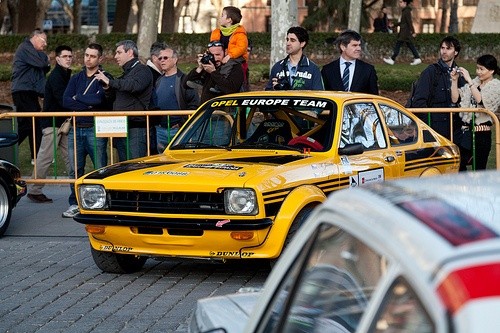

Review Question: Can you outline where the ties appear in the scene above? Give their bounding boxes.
[341,62,352,92]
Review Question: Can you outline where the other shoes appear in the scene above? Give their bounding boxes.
[31,159,55,165]
[27,192,53,202]
[409,58,422,65]
[383,57,395,65]
[186,78,204,90]
[62,204,80,218]
[209,84,222,92]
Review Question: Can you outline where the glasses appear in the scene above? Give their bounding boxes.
[207,41,223,47]
[158,56,169,60]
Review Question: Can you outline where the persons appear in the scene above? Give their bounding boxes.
[152,48,199,154]
[27,45,74,204]
[451,54,500,171]
[321,30,378,95]
[373,6,388,33]
[62,43,117,218]
[183,39,245,147]
[383,0,422,66]
[94,39,154,162]
[263,26,325,120]
[146,41,170,81]
[404,35,470,172]
[209,6,252,94]
[9,28,56,166]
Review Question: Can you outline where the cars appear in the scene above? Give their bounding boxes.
[188,168,500,333]
[0,131,27,239]
[72,89,461,273]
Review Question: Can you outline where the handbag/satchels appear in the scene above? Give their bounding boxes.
[57,118,73,137]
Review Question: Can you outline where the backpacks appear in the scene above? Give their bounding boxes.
[405,62,449,107]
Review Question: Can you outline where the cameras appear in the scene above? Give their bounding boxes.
[201,53,215,65]
[455,68,463,73]
